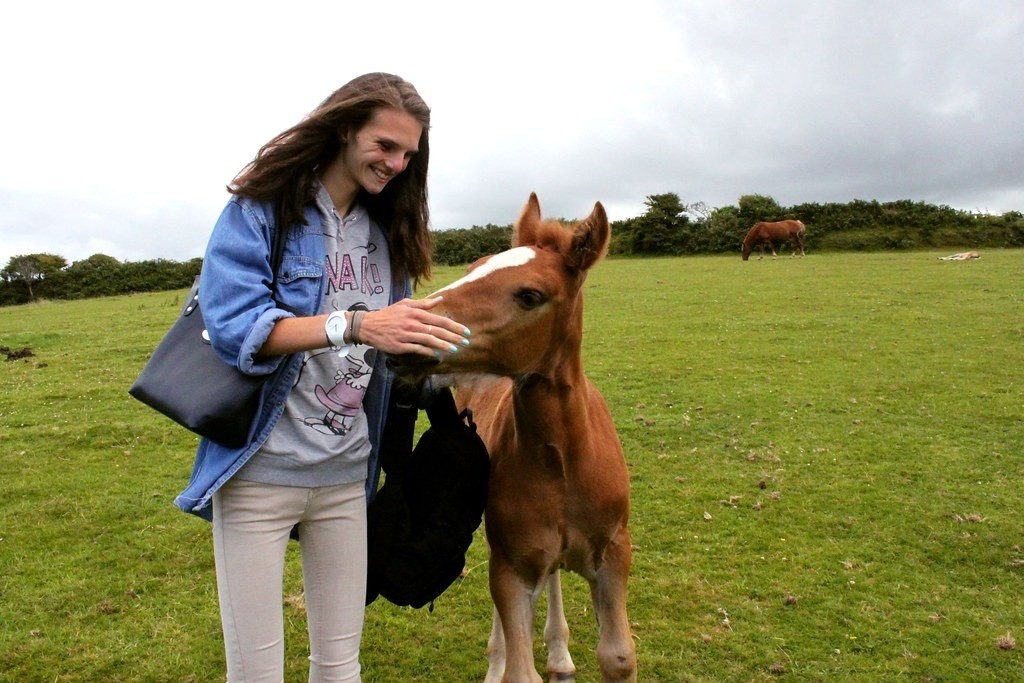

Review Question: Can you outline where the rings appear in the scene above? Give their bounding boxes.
[428,324,432,334]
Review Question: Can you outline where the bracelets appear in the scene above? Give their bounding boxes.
[348,310,366,347]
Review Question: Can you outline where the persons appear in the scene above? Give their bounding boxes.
[173,72,471,682]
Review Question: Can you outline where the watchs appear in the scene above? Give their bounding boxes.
[325,310,352,348]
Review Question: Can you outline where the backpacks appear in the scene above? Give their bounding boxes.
[367,374,490,610]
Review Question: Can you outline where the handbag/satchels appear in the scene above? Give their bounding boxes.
[127,198,310,451]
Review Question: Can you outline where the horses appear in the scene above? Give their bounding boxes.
[739,219,807,261]
[384,193,638,683]
